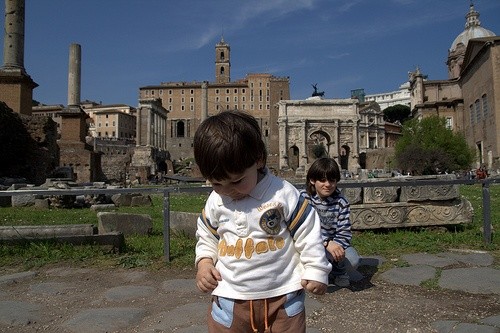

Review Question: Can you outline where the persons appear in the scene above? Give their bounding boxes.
[300,158,359,286]
[454,166,473,180]
[194,110,332,333]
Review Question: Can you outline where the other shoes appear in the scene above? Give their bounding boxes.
[336,273,350,286]
